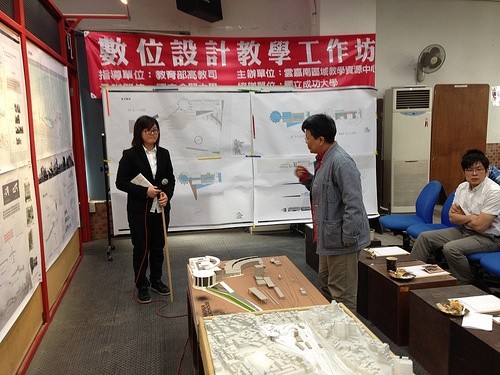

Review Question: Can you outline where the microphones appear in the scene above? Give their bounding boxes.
[146,178,169,199]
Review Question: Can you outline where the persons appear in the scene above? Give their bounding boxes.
[39,155,71,179]
[116,115,175,302]
[408,149,500,284]
[294,113,372,316]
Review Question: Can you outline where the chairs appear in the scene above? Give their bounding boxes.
[406,190,458,269]
[378,181,443,250]
[467,251,500,279]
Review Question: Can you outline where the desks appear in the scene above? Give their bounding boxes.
[186,254,331,375]
[304,223,381,274]
[409,284,499,375]
[357,246,456,346]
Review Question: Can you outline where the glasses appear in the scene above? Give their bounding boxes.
[466,167,484,173]
[145,129,160,134]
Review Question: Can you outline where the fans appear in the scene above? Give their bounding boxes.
[416,44,447,82]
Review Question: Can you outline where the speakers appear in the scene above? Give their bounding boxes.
[175,0,223,23]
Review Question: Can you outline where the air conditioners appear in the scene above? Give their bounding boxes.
[382,86,433,214]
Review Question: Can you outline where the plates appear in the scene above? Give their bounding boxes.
[439,304,470,316]
[390,273,416,281]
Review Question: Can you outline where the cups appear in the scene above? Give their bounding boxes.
[370,229,375,240]
[386,257,398,272]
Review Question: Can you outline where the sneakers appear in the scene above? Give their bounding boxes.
[151,283,171,295]
[137,287,152,304]
